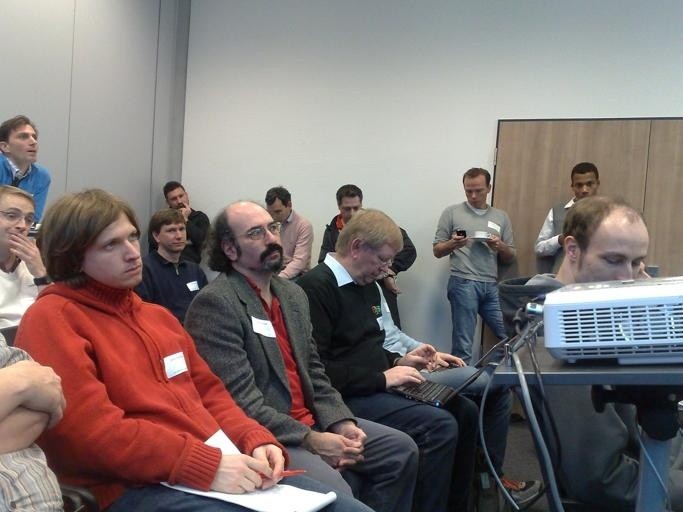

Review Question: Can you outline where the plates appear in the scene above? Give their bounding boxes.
[468,237,493,243]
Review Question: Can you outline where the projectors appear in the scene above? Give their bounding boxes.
[542,276,683,366]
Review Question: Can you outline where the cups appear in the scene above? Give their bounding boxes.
[474,231,491,238]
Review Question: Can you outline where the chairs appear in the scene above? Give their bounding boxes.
[0,326,100,512]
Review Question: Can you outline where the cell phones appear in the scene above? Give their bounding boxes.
[455,229,467,238]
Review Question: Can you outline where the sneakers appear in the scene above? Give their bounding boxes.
[498,475,545,510]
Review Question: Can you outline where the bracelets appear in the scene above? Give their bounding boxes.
[34,274,52,285]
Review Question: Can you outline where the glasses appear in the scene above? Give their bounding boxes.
[234,220,281,241]
[0,211,35,229]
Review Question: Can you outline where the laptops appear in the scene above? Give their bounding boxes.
[418,336,510,374]
[385,364,488,407]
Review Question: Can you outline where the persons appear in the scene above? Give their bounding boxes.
[513,195,683,512]
[316,185,418,330]
[12,189,376,511]
[148,181,211,266]
[1,115,51,222]
[294,208,482,512]
[1,331,70,511]
[433,168,516,366]
[134,209,209,325]
[534,162,601,273]
[264,186,313,280]
[375,281,549,511]
[183,200,418,512]
[1,185,54,328]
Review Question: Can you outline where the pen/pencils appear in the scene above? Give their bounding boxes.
[257,469,307,478]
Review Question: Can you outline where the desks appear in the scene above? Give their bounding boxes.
[493,336,683,511]
